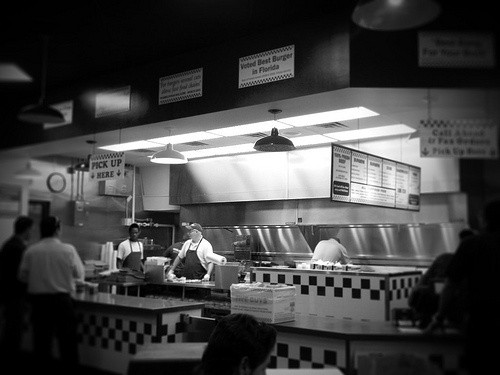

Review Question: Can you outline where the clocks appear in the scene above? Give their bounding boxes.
[47,173,67,193]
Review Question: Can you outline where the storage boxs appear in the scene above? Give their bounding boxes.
[215,265,239,289]
[230,282,296,324]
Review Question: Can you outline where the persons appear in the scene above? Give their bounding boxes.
[310,237,354,266]
[116,223,145,273]
[165,222,214,281]
[0,217,35,375]
[408,199,500,375]
[194,313,278,375]
[19,215,86,375]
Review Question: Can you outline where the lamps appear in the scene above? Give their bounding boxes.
[150,126,188,164]
[253,109,296,152]
[12,146,43,180]
[17,57,65,125]
[351,0,440,30]
[75,140,97,171]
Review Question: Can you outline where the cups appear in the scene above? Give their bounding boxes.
[100,241,117,294]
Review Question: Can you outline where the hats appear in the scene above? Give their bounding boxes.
[186,223,204,232]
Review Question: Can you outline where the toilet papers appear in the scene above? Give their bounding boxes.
[206,253,227,266]
